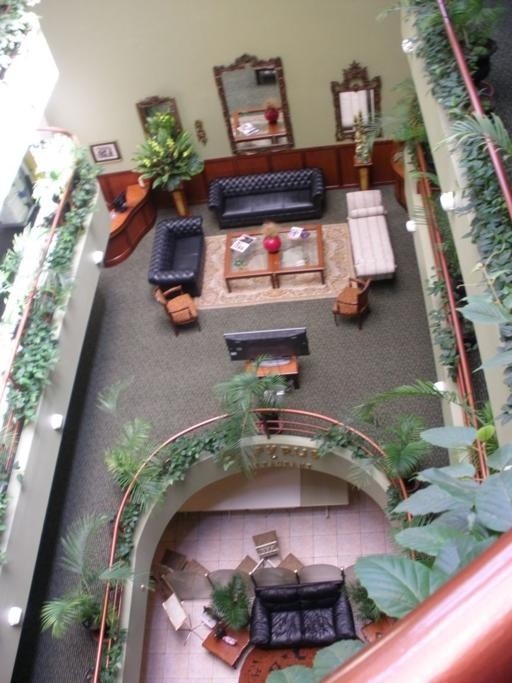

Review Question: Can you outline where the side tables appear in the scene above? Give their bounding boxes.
[201,620,250,670]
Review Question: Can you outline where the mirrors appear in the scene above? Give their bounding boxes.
[213,55,305,149]
[332,60,381,141]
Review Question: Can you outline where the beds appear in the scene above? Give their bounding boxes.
[346,189,397,283]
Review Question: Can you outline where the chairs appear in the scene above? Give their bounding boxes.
[152,284,201,335]
[332,276,373,328]
[157,530,305,577]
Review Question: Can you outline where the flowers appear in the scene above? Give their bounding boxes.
[132,111,204,191]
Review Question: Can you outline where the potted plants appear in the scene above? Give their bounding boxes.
[363,416,431,490]
[38,508,146,640]
[214,353,286,481]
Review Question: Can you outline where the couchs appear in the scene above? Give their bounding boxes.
[250,580,356,649]
[148,216,205,297]
[208,167,325,229]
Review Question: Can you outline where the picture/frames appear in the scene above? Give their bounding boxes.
[90,142,120,163]
[136,96,183,147]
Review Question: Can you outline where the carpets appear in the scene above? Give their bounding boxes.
[192,223,357,310]
[239,645,324,683]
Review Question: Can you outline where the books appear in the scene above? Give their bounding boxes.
[237,123,260,136]
[230,234,256,252]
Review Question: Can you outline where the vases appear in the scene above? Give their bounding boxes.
[263,236,282,253]
[173,189,189,218]
[265,109,278,124]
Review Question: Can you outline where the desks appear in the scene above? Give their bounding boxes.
[245,356,300,390]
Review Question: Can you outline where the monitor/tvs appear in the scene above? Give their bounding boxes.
[113,192,128,213]
[224,327,310,367]
